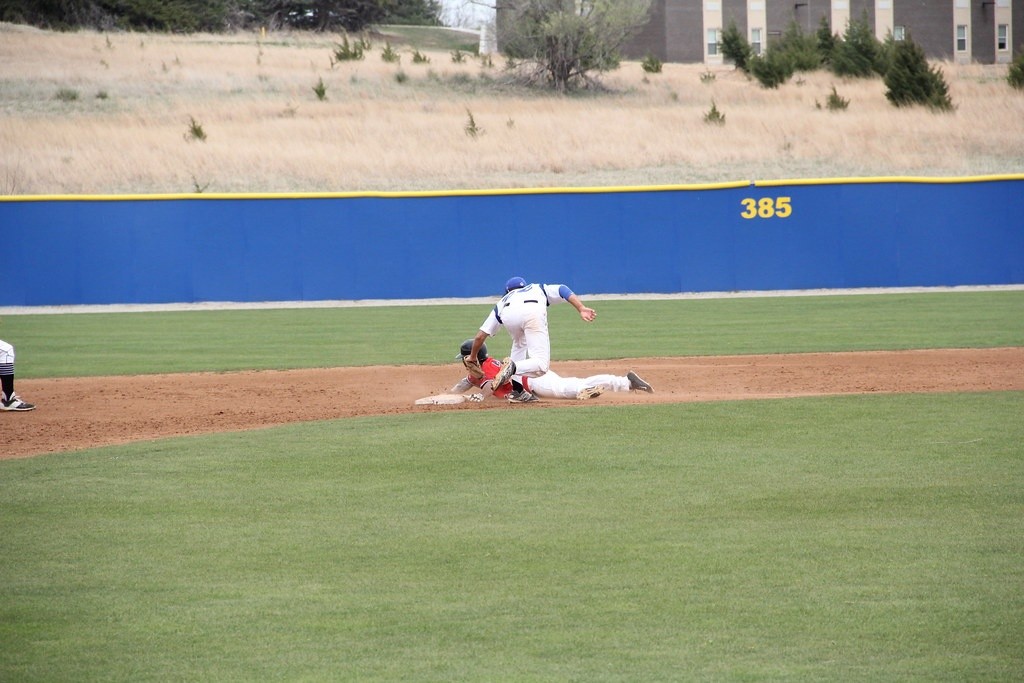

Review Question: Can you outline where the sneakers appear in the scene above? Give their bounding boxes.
[508,389,540,403]
[578,384,606,401]
[1,392,36,411]
[626,370,653,393]
[491,358,516,390]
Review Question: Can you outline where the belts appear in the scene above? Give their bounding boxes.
[502,300,542,305]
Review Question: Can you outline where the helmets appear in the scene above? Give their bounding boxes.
[502,277,527,297]
[455,339,489,361]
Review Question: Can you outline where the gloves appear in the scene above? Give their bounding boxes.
[463,393,484,402]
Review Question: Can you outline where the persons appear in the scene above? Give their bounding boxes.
[465,276,597,405]
[441,339,656,404]
[1,338,37,413]
